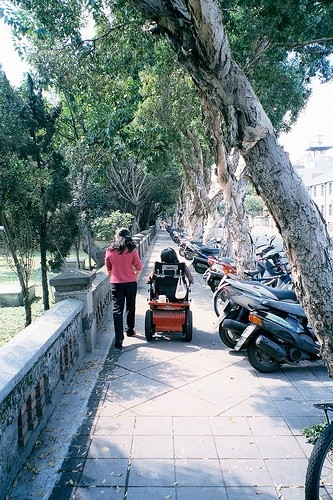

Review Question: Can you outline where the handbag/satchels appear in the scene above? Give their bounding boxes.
[174,278,187,299]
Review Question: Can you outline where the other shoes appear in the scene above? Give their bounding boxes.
[126,330,136,337]
[113,342,126,349]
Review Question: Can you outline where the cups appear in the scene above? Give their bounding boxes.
[159,295,169,303]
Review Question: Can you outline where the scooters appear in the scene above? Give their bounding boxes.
[164,224,300,351]
[234,299,322,373]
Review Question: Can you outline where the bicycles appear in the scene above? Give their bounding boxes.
[304,402,333,500]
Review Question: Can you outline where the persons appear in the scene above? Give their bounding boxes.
[105,228,142,348]
[147,247,194,286]
